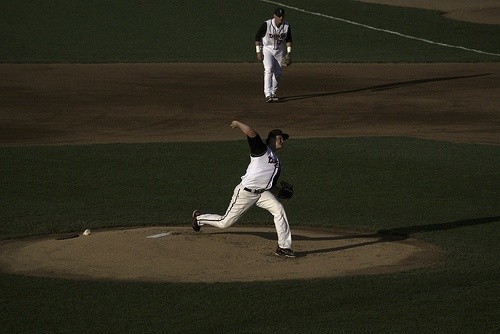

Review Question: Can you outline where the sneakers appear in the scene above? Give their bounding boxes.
[191,209,201,232]
[276,247,296,259]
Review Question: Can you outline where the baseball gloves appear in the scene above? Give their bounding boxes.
[272,181,294,199]
[281,54,292,67]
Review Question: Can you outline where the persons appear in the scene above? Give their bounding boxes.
[192,120,295,257]
[255,8,293,102]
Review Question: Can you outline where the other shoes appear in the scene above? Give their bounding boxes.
[264,96,279,102]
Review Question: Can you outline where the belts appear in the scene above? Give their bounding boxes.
[244,187,266,194]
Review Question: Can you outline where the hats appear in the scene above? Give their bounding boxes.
[274,8,285,16]
[268,129,290,142]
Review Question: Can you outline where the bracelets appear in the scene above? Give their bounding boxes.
[256,46,260,53]
[287,47,291,53]
